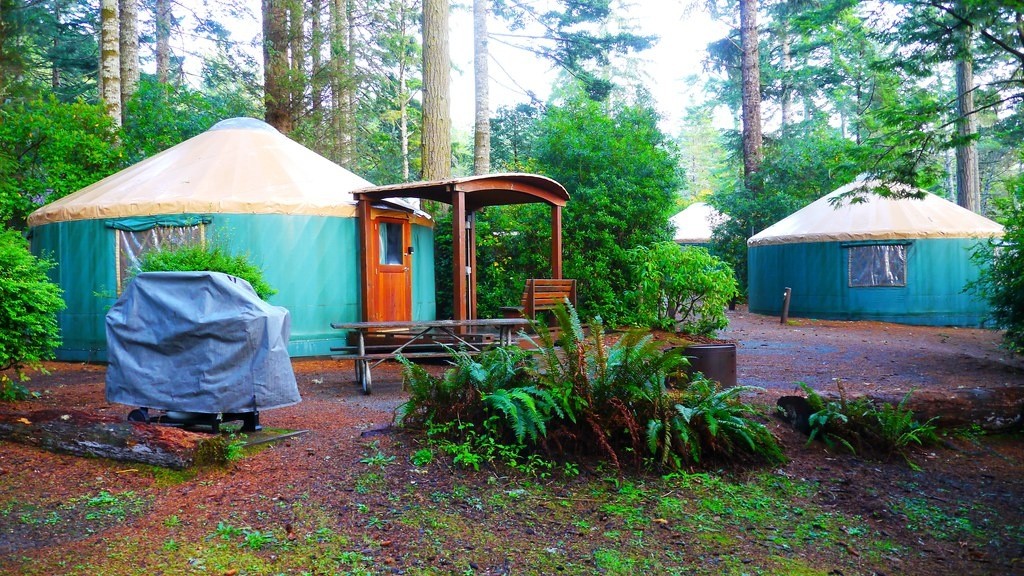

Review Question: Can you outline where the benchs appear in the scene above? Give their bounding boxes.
[329,341,520,383]
[497,277,577,334]
[331,351,491,396]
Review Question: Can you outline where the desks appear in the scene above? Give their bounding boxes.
[330,318,538,389]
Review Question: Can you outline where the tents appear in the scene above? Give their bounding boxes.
[27,117,438,360]
[667,202,744,280]
[747,180,1014,330]
